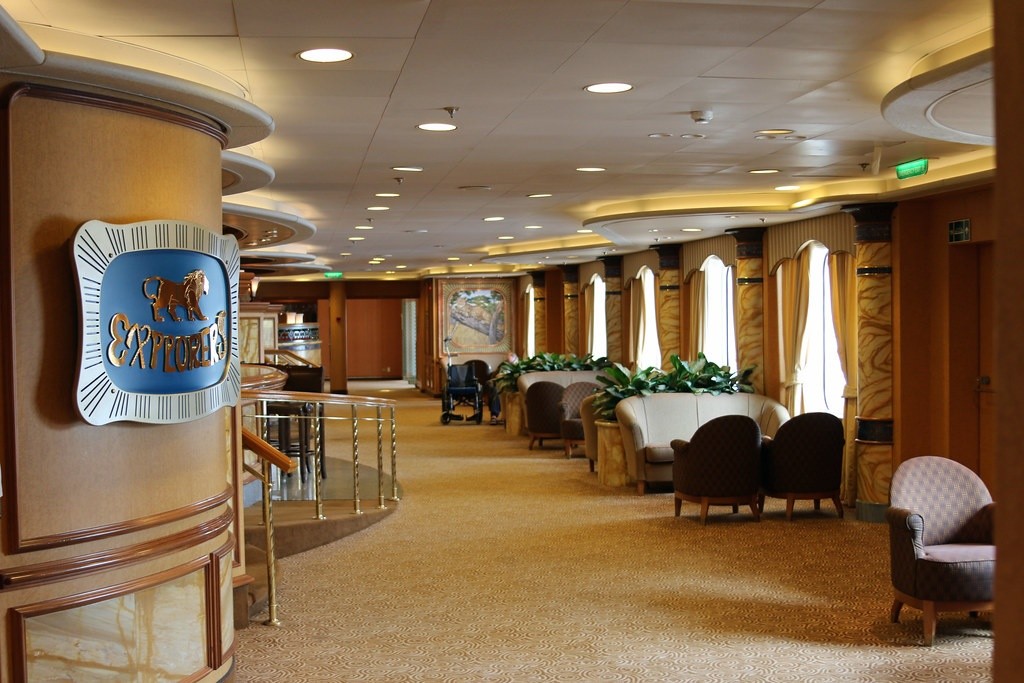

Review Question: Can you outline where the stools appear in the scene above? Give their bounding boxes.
[267,366,327,483]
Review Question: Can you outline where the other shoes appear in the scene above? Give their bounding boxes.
[489,418,497,425]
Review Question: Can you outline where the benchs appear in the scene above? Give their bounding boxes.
[615,393,791,497]
[517,371,616,429]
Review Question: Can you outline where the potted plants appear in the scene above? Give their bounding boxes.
[592,363,667,488]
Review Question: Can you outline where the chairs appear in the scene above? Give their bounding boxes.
[580,391,607,473]
[464,360,491,380]
[887,456,996,647]
[557,382,602,459]
[525,381,565,451]
[758,412,846,523]
[670,415,762,527]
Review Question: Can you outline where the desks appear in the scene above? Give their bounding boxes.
[240,364,288,392]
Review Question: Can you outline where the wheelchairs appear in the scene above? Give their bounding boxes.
[440,362,484,425]
